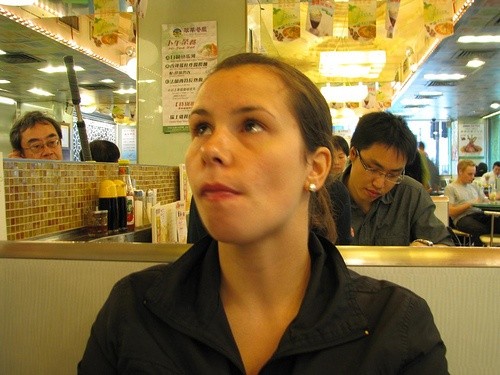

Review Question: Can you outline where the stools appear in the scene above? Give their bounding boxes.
[450,229,475,247]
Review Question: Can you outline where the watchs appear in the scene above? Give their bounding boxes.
[413,239,433,247]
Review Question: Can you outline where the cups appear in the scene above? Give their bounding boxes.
[88,210,108,236]
[310,9,322,31]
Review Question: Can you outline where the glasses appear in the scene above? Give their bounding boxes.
[21,139,60,153]
[356,146,405,184]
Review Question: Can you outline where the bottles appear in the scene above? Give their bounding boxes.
[118,159,135,229]
[134,189,154,227]
[112,179,128,231]
[97,179,119,233]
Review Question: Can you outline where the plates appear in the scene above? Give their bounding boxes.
[282,25,300,41]
[196,50,217,61]
[357,23,376,40]
[433,21,454,37]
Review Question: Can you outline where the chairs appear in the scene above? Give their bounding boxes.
[479,210,500,248]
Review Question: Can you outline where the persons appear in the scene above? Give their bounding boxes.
[318,112,456,249]
[443,160,500,246]
[328,133,349,178]
[79,139,122,163]
[74,55,450,375]
[8,111,64,164]
[404,134,443,196]
